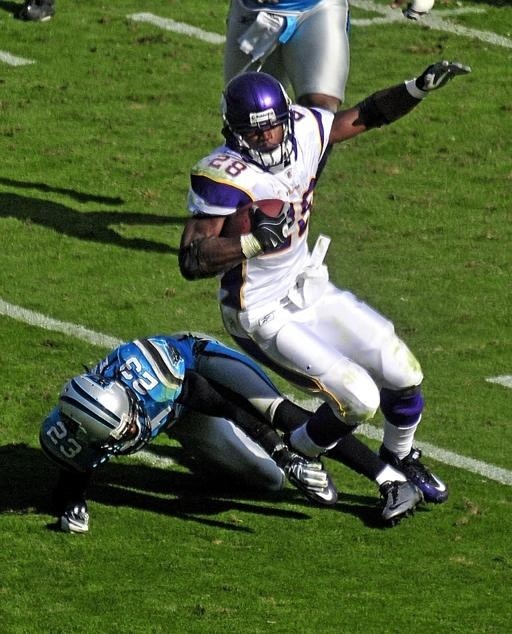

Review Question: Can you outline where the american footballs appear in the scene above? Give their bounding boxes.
[219,199,285,237]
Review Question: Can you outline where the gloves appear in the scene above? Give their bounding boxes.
[247,200,310,257]
[415,59,474,92]
[59,504,89,534]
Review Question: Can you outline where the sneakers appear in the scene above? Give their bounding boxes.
[378,440,449,503]
[273,429,339,506]
[379,479,424,523]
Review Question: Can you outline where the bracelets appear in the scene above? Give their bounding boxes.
[404,77,429,100]
[238,232,262,259]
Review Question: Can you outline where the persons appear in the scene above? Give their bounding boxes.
[220,0,439,186]
[178,58,473,504]
[44,331,422,535]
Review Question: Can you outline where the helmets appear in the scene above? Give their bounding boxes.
[57,373,138,447]
[219,69,293,138]
